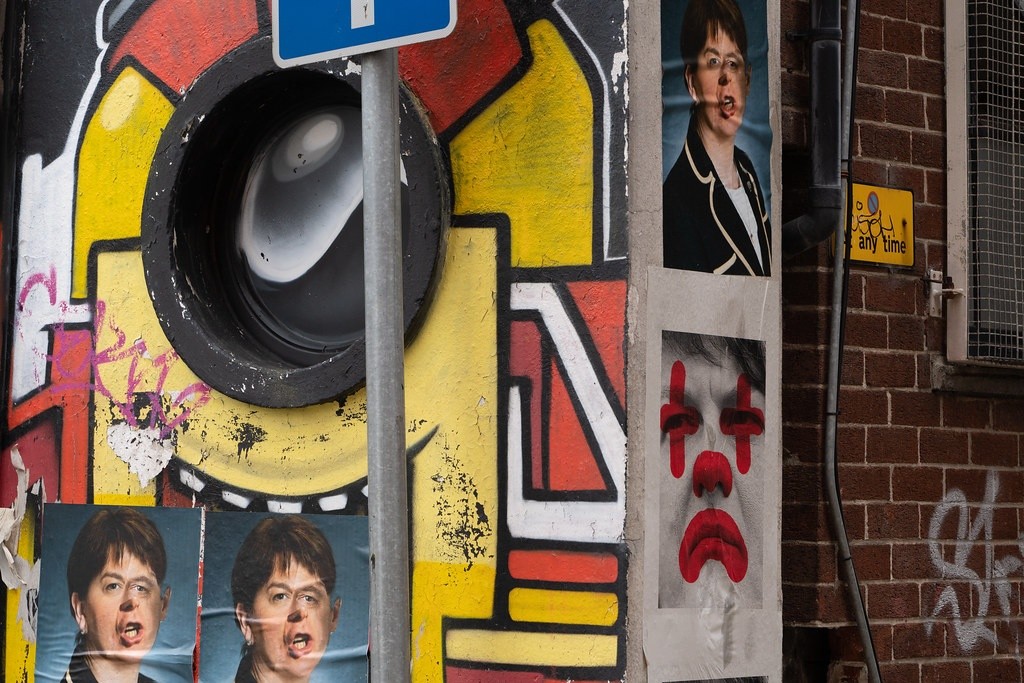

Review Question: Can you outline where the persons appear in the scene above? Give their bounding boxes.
[230,514,341,683]
[660,327,763,610]
[663,1,771,277]
[59,505,172,683]
